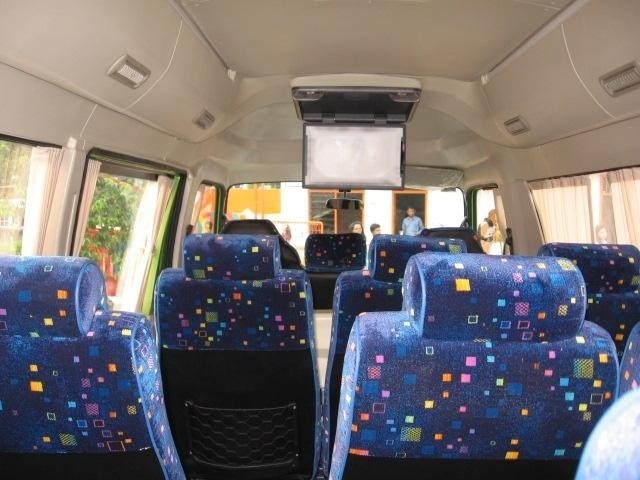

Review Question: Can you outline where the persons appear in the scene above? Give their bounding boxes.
[401,207,425,236]
[348,221,368,271]
[478,208,504,256]
[370,223,381,237]
[595,224,610,244]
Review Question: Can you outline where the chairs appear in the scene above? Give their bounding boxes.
[1,221,638,478]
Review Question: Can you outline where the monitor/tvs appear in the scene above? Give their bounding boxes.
[301,122,407,191]
